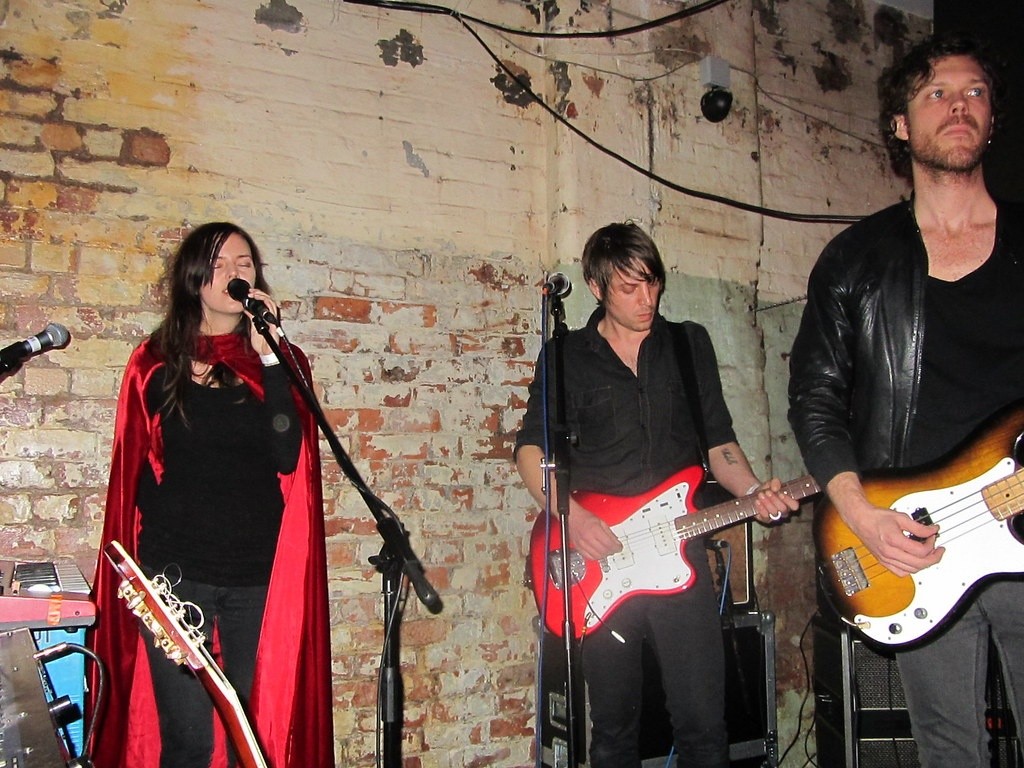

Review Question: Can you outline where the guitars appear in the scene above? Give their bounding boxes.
[527,462,824,642]
[103,538,274,768]
[809,405,1024,657]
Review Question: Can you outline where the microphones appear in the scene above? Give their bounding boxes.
[0,323,69,363]
[542,272,570,294]
[227,278,276,324]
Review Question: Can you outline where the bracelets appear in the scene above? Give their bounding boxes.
[259,352,280,367]
[744,483,760,496]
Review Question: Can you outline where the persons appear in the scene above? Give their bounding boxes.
[514,219,799,768]
[786,30,1024,768]
[82,222,335,768]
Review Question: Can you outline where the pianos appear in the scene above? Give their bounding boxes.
[0,557,98,636]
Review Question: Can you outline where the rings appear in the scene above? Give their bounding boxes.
[768,510,784,520]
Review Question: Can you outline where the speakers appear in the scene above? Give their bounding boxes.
[813,616,1024,768]
